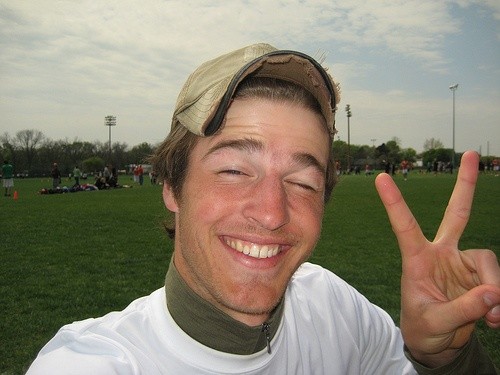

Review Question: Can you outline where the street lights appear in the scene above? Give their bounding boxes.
[449,83,459,167]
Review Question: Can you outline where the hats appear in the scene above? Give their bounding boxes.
[173,42,345,149]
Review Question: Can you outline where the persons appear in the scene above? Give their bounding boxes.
[49,162,158,189]
[21,41,500,375]
[1,159,14,197]
[334,157,500,181]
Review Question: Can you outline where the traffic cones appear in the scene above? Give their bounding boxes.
[14,191,18,200]
